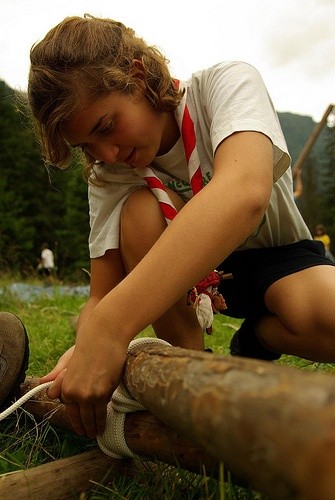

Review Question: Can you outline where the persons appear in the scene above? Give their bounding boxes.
[26,13,335,438]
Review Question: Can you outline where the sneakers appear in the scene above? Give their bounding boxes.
[1,312,29,434]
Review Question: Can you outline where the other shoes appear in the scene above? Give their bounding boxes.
[231,312,283,361]
